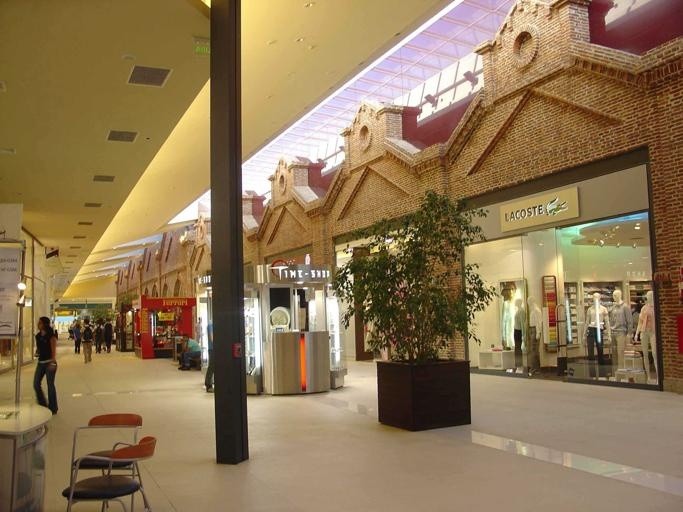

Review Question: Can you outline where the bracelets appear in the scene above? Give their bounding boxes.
[48,363,57,366]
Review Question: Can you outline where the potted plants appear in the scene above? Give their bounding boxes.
[329,186,494,433]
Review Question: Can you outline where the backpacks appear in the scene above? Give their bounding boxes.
[82,325,93,341]
[95,324,102,339]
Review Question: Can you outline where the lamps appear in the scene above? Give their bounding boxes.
[16,295,25,307]
[19,277,26,291]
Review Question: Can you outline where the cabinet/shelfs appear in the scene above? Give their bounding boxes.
[564,277,653,345]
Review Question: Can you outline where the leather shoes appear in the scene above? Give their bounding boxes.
[181,366,191,370]
[206,385,213,393]
[178,366,184,369]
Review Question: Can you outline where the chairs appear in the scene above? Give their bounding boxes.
[60,435,155,511]
[67,412,149,510]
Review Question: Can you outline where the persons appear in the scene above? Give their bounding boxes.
[32,316,58,415]
[73,323,82,354]
[103,317,114,353]
[178,334,201,370]
[582,291,612,382]
[633,291,659,385]
[512,298,526,373]
[630,301,643,331]
[80,318,96,364]
[202,321,216,393]
[194,316,205,344]
[608,288,635,379]
[92,320,104,354]
[523,296,542,376]
